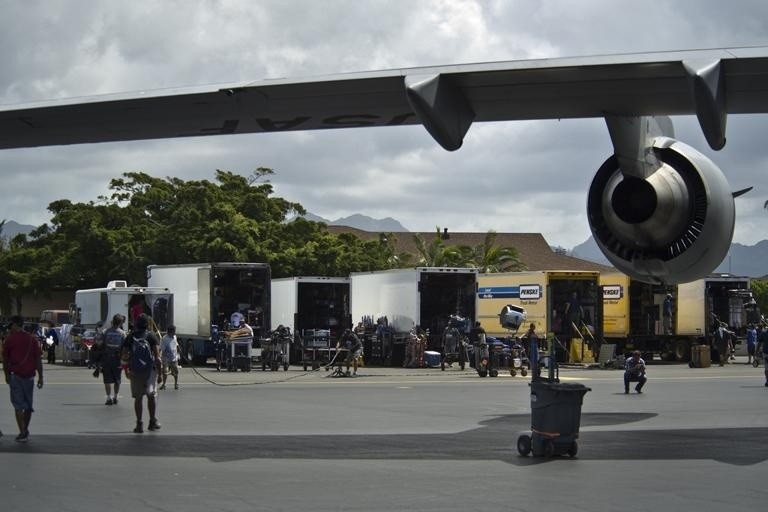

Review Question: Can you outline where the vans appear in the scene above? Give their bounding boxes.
[41,309,75,325]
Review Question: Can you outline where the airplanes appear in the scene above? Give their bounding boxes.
[0,44,768,290]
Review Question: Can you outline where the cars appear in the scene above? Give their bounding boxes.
[0,322,48,356]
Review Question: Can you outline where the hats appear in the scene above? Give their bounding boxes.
[7,313,25,325]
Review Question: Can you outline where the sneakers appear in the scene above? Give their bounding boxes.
[15,431,29,441]
[133,420,144,433]
[112,398,118,404]
[624,389,630,396]
[175,383,179,390]
[635,387,642,393]
[345,370,359,377]
[148,417,161,431]
[160,383,167,390]
[105,399,112,405]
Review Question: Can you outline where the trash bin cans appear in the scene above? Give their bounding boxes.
[516,378,592,459]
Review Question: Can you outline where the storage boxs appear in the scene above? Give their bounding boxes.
[422,348,443,367]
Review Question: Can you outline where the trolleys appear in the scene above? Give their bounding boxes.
[752,339,765,368]
[217,336,252,373]
[516,332,591,459]
[261,337,289,373]
[303,326,331,373]
[481,342,532,378]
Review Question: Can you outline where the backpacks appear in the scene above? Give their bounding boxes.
[129,331,156,371]
[103,327,124,361]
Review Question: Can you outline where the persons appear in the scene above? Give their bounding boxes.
[130,299,143,324]
[158,324,183,390]
[70,319,86,336]
[622,349,647,393]
[3,315,43,443]
[663,293,673,335]
[562,289,584,337]
[27,323,58,364]
[96,315,126,406]
[122,311,164,432]
[552,309,562,332]
[718,317,768,386]
